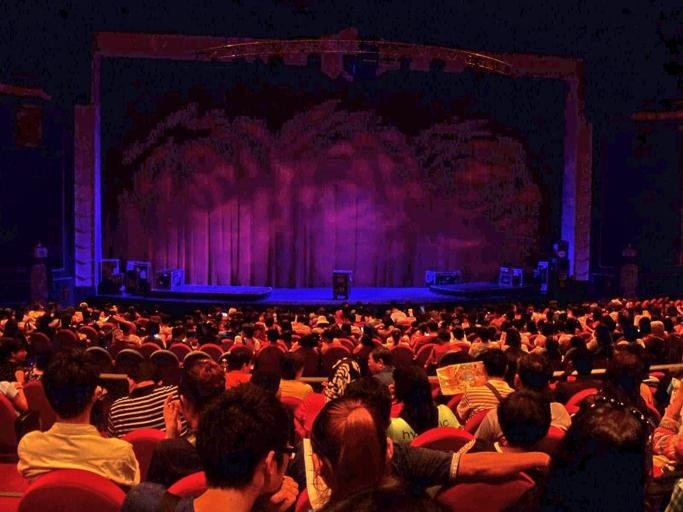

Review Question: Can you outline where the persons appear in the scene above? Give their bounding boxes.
[562,352,606,403]
[15,349,140,490]
[543,396,651,511]
[381,367,463,437]
[479,355,564,446]
[295,355,365,432]
[457,352,520,422]
[1,383,32,422]
[344,376,414,444]
[217,349,257,389]
[275,353,320,400]
[570,345,663,431]
[650,375,683,479]
[418,293,683,373]
[0,338,29,393]
[107,360,190,438]
[124,384,300,512]
[252,367,278,400]
[306,396,451,511]
[146,354,226,486]
[365,352,402,375]
[290,375,553,492]
[472,395,564,496]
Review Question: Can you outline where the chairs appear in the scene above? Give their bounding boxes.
[0,464,37,512]
[24,379,57,433]
[102,374,129,398]
[16,468,125,512]
[1,391,37,454]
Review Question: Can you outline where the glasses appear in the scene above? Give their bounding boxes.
[280,443,298,461]
